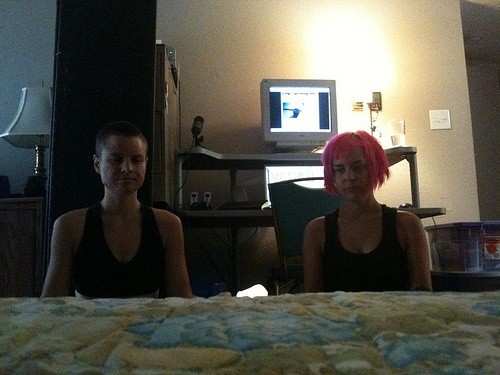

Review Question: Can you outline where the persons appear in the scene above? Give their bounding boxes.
[38,121,196,299]
[300,130,435,292]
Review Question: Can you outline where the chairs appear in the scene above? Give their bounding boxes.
[268,177,343,295]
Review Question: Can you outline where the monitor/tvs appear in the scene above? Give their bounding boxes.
[260,79,338,153]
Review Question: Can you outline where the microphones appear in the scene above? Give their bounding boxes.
[191,116,204,137]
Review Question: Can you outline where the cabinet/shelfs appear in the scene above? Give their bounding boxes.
[1,198,47,299]
[175,145,447,300]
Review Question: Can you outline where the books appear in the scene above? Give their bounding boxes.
[218,199,271,211]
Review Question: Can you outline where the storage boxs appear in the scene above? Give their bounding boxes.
[424,220,499,276]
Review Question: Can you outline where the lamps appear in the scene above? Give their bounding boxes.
[1,80,52,196]
